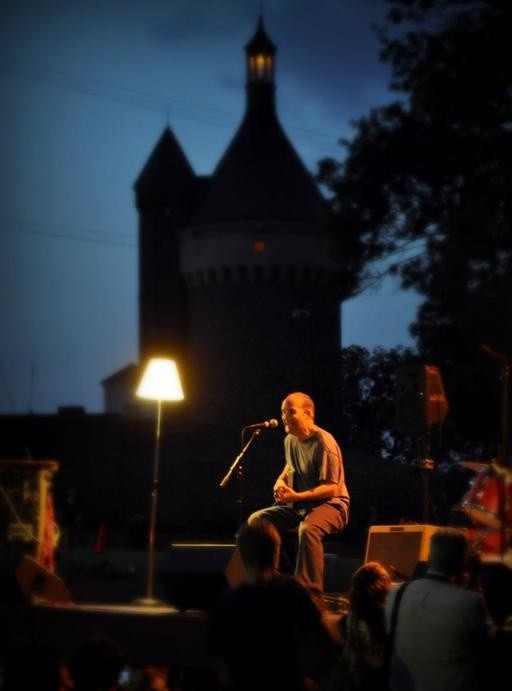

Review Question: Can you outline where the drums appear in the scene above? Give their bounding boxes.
[459,467,512,529]
[462,526,512,562]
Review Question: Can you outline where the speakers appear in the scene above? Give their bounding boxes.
[172,545,252,612]
[14,555,70,607]
[395,365,449,434]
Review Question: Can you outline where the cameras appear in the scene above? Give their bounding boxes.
[117,668,143,691]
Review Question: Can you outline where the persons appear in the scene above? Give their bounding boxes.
[0,519,512,691]
[246,391,350,592]
[461,448,512,568]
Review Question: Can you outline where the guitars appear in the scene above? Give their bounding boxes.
[274,468,294,509]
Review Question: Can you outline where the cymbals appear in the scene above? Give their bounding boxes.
[457,461,489,473]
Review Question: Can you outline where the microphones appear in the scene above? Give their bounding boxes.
[249,418,279,429]
